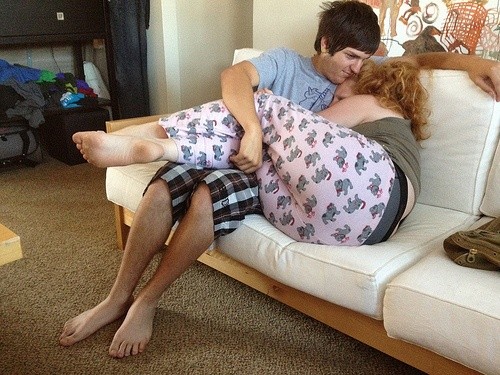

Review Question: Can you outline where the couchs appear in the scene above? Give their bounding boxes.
[102,45,500,375]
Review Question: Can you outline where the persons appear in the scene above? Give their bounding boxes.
[72,60,431,246]
[58,0,500,358]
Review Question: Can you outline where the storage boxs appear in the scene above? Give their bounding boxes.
[43,108,112,166]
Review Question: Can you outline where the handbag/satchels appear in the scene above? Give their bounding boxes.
[443,217,500,271]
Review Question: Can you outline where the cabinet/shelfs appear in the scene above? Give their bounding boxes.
[0,0,119,131]
